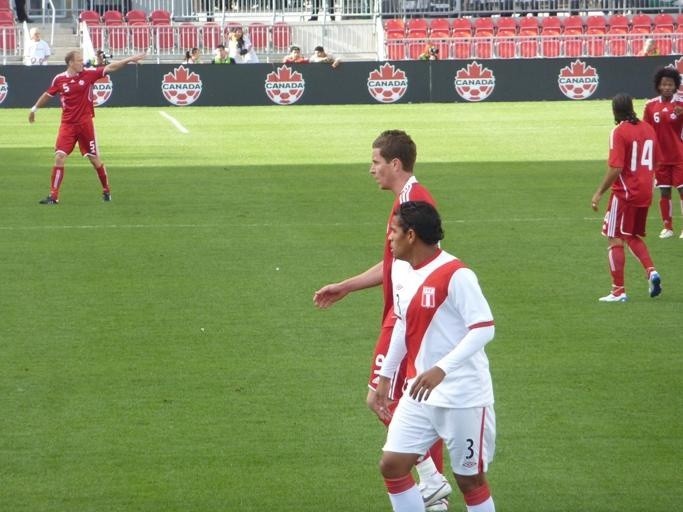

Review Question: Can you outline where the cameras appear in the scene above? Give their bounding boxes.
[429,47,439,54]
[103,54,112,59]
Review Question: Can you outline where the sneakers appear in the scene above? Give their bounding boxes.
[659,228,674,239]
[424,497,449,512]
[418,476,453,508]
[102,189,112,203]
[38,196,60,206]
[647,270,663,298]
[599,293,628,304]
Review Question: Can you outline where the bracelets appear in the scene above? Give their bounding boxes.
[30,106,37,112]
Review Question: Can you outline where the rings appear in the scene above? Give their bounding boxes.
[592,203,595,207]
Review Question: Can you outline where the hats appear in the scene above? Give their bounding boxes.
[30,28,40,37]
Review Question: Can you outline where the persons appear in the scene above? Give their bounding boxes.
[311,130,451,510]
[589,93,662,303]
[22,27,52,66]
[284,46,340,68]
[419,45,438,60]
[373,201,495,511]
[637,38,660,57]
[182,27,260,64]
[642,69,683,240]
[28,51,148,203]
[85,51,110,65]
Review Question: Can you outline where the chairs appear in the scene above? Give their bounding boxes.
[0,0,682,69]
